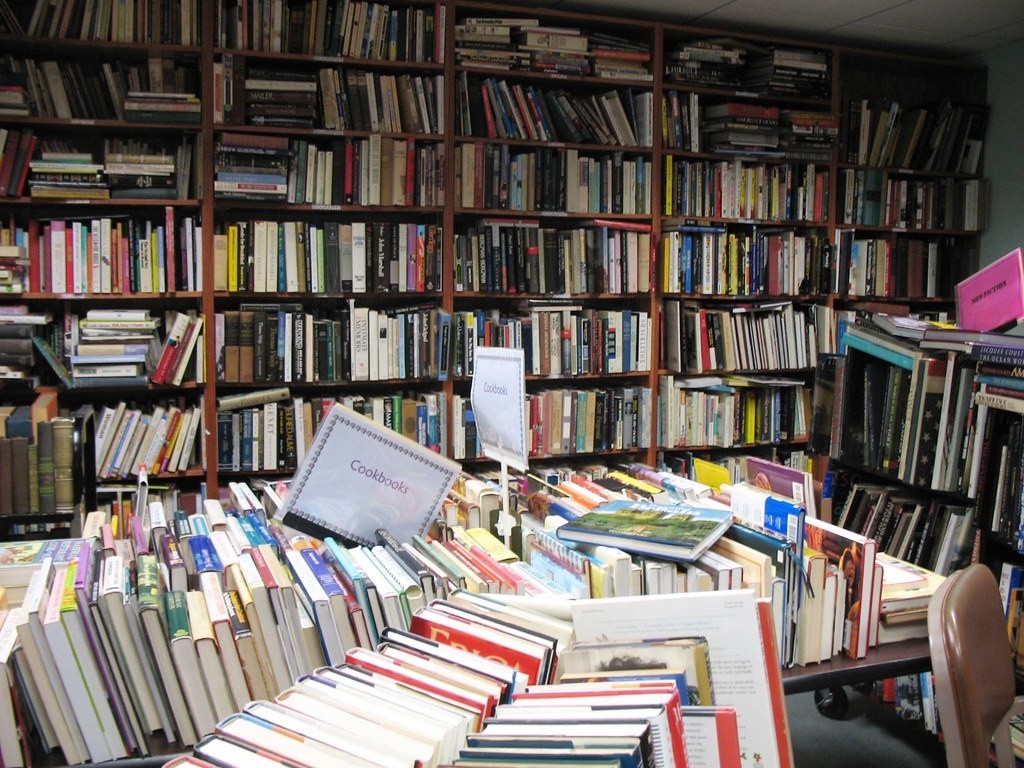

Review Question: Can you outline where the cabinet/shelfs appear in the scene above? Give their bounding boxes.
[0,0,983,530]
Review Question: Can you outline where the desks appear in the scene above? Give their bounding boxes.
[775,636,932,696]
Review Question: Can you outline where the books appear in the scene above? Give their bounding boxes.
[557,499,736,562]
[0,0,1024,768]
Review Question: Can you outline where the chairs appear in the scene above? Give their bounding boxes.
[927,564,1024,768]
